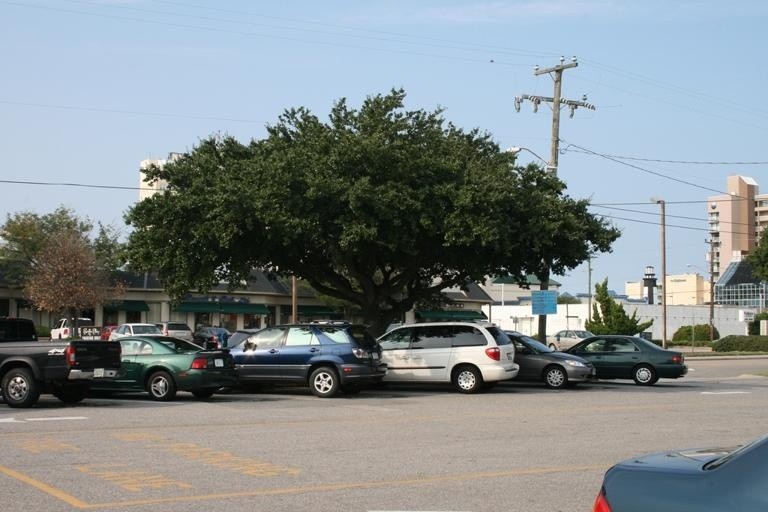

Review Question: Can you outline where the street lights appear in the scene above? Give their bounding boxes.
[650,197,669,349]
[686,264,715,342]
[505,147,559,347]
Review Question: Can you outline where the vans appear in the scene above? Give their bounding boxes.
[50,316,92,341]
[156,322,193,342]
[213,324,388,397]
[375,322,520,393]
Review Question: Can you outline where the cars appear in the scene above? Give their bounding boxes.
[83,336,233,400]
[101,325,119,341]
[109,323,165,342]
[220,328,260,347]
[308,319,353,325]
[592,430,768,511]
[548,330,597,352]
[502,330,598,389]
[557,334,689,386]
[195,326,231,349]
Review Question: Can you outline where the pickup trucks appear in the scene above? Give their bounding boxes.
[1,317,122,408]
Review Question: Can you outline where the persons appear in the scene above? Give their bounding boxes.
[194,324,212,349]
